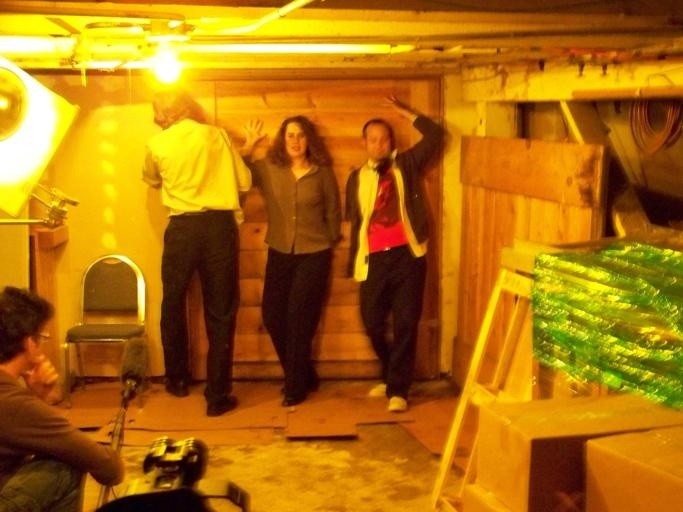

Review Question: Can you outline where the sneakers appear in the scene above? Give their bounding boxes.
[281,376,320,393]
[205,396,238,416]
[164,379,188,397]
[281,394,306,407]
[368,383,387,398]
[388,396,408,413]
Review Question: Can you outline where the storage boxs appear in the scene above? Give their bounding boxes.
[463,390,682,512]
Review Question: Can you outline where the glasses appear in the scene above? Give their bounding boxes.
[38,331,52,343]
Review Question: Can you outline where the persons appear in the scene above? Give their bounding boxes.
[0,285,126,512]
[143,89,256,418]
[238,116,344,410]
[337,92,449,414]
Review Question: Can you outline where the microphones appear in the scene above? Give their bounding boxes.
[118,337,148,402]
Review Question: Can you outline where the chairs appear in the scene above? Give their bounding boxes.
[62,253,148,410]
